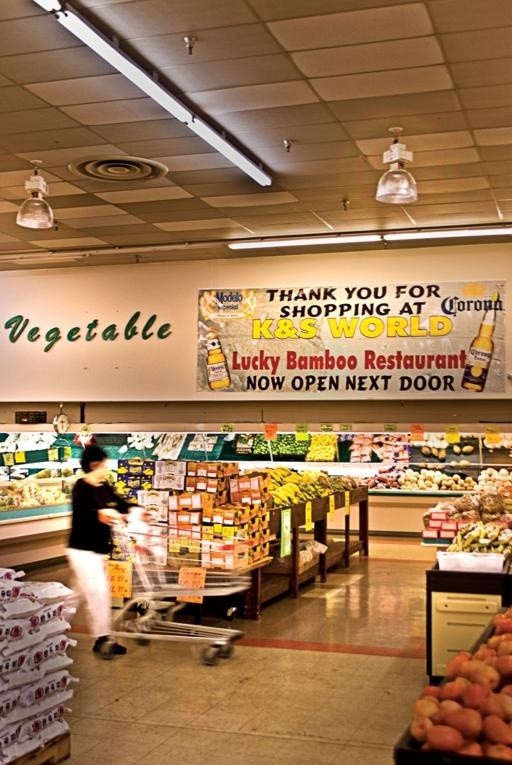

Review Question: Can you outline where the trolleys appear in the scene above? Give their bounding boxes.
[103,512,252,666]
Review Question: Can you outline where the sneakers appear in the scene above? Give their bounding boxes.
[93,640,127,656]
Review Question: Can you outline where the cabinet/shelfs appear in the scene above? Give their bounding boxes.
[1,569,80,765]
[393,497,512,765]
[117,483,371,619]
[1,434,511,537]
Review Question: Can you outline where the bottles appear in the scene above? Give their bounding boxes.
[206,325,231,391]
[460,307,496,394]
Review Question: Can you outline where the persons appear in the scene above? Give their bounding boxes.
[57,443,154,659]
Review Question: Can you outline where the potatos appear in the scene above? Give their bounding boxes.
[399,469,477,490]
[421,444,474,460]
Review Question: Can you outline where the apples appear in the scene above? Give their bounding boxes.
[410,608,512,761]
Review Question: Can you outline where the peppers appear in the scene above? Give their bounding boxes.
[252,434,338,461]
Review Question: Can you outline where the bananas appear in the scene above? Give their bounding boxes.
[245,467,334,507]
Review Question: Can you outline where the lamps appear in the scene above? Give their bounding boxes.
[374,136,420,206]
[35,1,63,14]
[184,116,273,187]
[226,224,512,254]
[14,159,54,230]
[55,11,194,125]
